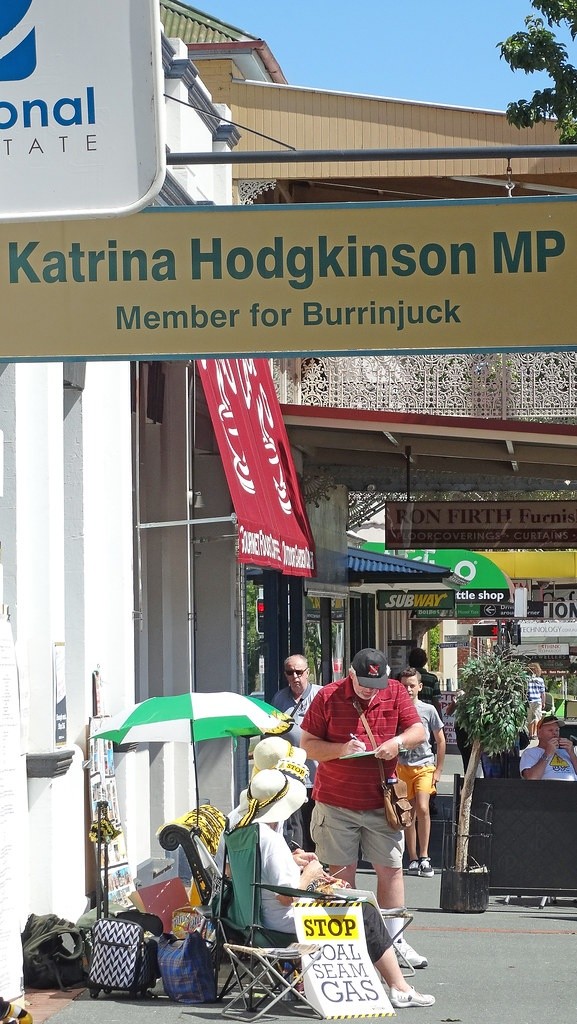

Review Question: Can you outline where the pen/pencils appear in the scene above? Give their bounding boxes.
[350,733,359,741]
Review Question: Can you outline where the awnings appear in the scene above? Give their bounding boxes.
[139,359,318,577]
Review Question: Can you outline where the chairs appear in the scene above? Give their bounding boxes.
[159,802,414,1023]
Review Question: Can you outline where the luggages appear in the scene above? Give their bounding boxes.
[86,910,164,999]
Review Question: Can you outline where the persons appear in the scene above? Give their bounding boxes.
[396,648,443,815]
[270,654,324,852]
[526,663,545,740]
[299,648,428,969]
[238,769,435,1008]
[446,696,473,774]
[216,736,307,875]
[520,716,577,781]
[395,668,446,877]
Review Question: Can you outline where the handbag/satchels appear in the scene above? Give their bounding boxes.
[158,929,218,1005]
[380,778,413,831]
[22,913,91,991]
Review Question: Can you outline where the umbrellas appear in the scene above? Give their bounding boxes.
[88,692,282,826]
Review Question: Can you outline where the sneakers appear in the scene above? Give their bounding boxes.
[394,938,428,968]
[419,857,434,877]
[389,985,435,1007]
[407,860,419,876]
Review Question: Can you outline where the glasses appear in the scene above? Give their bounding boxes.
[284,667,308,675]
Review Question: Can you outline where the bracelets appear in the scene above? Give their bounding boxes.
[394,736,408,752]
[541,754,549,761]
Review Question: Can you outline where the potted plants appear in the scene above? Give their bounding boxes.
[441,646,536,913]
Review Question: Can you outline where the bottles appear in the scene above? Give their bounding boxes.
[282,962,293,1001]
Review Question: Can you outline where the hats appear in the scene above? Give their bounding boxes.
[537,716,566,731]
[238,768,307,828]
[351,648,388,689]
[252,737,307,777]
